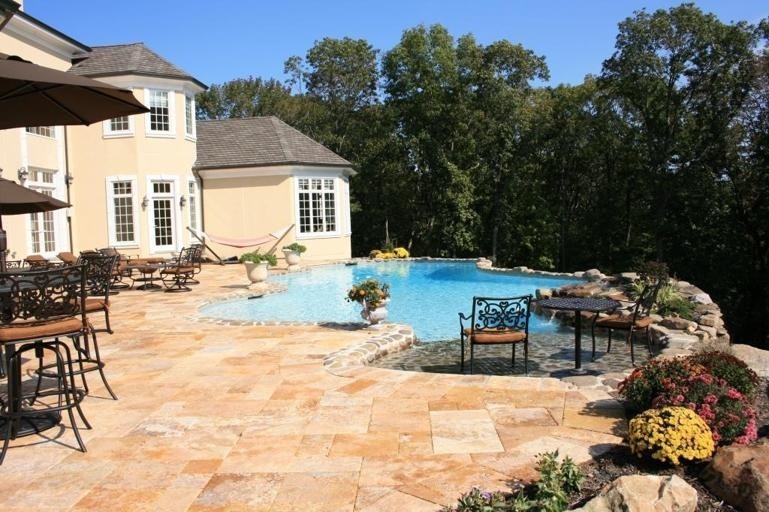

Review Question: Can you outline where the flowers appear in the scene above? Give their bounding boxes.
[344,279,392,306]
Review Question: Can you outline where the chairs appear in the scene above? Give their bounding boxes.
[95,244,203,293]
[590,281,661,368]
[1,250,120,469]
[457,293,533,375]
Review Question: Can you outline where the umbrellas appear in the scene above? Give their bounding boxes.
[0,175,73,217]
[0,58,152,131]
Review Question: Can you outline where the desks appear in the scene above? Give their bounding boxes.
[538,297,619,379]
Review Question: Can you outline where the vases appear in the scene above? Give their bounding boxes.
[359,297,390,326]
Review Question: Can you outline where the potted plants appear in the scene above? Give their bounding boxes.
[281,242,306,272]
[239,249,277,290]
